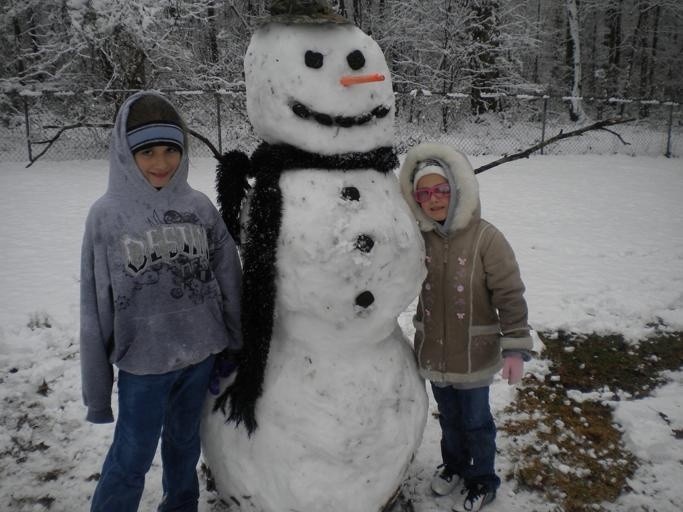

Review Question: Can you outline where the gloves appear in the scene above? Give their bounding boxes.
[208,354,238,394]
[502,351,524,385]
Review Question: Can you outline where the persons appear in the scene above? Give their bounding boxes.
[80,90,244,512]
[399,140,532,511]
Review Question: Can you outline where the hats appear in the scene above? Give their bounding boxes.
[126,94,185,154]
[411,158,451,194]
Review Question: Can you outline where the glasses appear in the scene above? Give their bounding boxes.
[414,182,451,203]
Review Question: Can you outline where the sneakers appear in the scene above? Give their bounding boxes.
[452,482,496,512]
[432,462,464,497]
